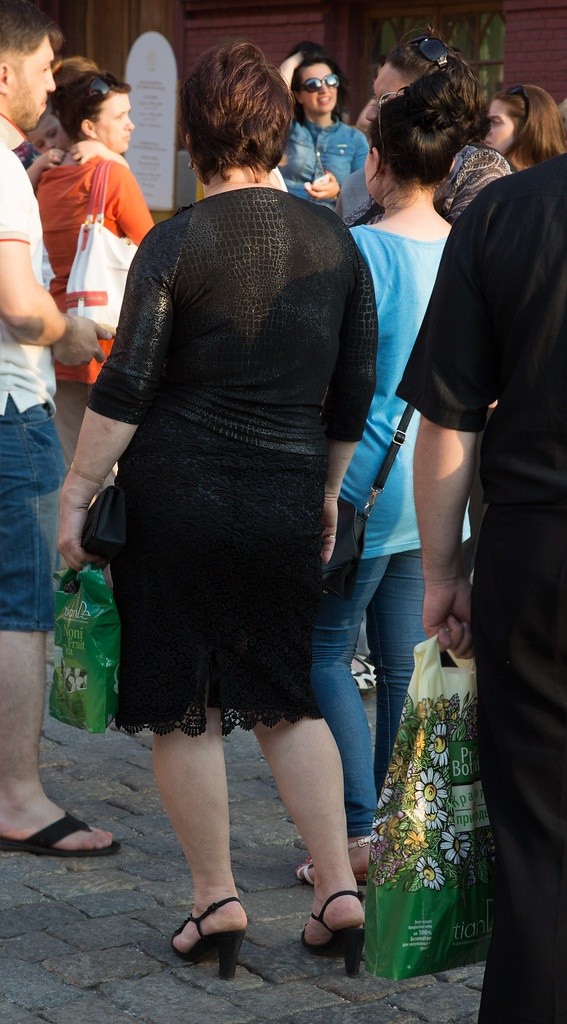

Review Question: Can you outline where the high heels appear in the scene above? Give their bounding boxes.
[302,891,363,976]
[171,897,245,980]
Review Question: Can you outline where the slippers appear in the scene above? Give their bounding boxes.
[0,812,122,858]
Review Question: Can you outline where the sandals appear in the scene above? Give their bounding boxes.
[294,834,371,885]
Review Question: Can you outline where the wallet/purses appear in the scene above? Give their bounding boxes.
[82,485,128,558]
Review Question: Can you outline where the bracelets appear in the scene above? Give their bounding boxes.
[71,461,105,484]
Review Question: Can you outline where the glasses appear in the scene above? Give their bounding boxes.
[377,91,408,174]
[406,34,450,70]
[85,72,119,106]
[506,85,530,107]
[300,73,340,91]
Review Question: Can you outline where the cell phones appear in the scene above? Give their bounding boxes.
[312,174,330,186]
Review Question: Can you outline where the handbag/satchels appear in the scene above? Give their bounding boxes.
[52,563,121,732]
[61,159,139,337]
[318,497,368,601]
[364,628,495,982]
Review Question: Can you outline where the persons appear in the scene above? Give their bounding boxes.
[0,0,121,857]
[277,33,567,887]
[58,42,378,979]
[13,56,155,591]
[395,151,567,1024]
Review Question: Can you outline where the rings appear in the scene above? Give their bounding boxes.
[325,534,336,538]
